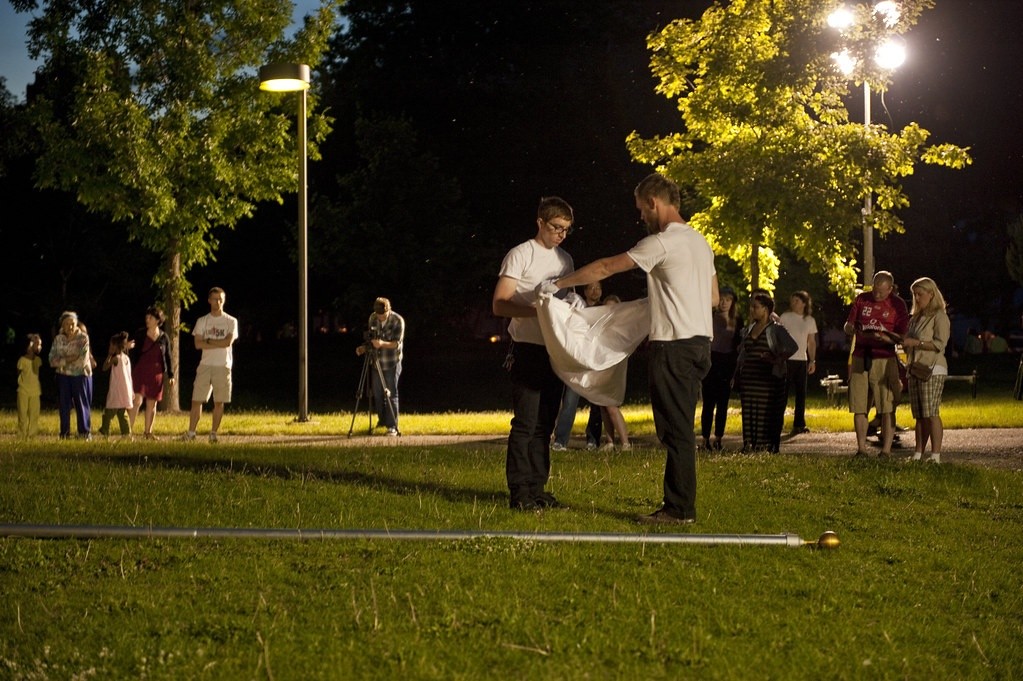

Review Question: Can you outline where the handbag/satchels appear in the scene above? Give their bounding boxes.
[910,361,933,381]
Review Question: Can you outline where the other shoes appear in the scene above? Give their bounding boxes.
[600,445,615,453]
[552,442,566,452]
[868,418,883,428]
[879,433,901,448]
[713,440,722,450]
[621,444,631,452]
[794,426,808,433]
[703,442,712,452]
[636,507,694,524]
[510,494,570,512]
[586,442,597,452]
[387,427,397,437]
[209,435,218,443]
[173,433,197,440]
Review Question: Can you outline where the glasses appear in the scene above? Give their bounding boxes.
[542,218,574,235]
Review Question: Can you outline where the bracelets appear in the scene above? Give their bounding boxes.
[205,338,210,343]
[810,360,816,363]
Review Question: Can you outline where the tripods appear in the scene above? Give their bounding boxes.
[348,343,401,437]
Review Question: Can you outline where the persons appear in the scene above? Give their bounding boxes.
[550,280,634,453]
[844,270,950,464]
[47,311,96,442]
[175,287,238,445]
[15,332,44,438]
[98,332,133,438]
[126,309,175,440]
[487,196,576,510]
[355,296,407,436]
[697,285,818,454]
[534,173,720,524]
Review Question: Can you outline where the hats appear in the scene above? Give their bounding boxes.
[719,286,738,303]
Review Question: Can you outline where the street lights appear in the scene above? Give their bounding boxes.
[258,62,312,424]
[829,0,908,293]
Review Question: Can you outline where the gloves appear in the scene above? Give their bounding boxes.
[534,277,560,306]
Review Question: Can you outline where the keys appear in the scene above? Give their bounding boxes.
[502,353,515,371]
